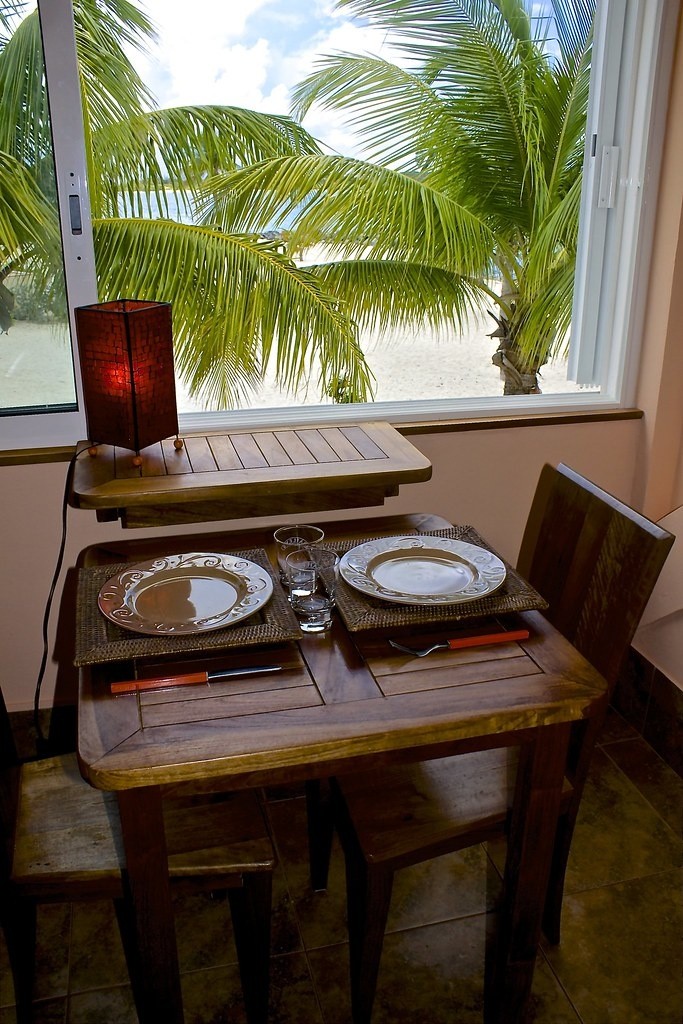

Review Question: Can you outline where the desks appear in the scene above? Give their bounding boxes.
[76,420,607,1024]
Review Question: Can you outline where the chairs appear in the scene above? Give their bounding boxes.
[308,462,674,1022]
[0,695,272,1024]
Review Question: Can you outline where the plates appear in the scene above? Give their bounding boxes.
[97,552,273,635]
[339,535,508,604]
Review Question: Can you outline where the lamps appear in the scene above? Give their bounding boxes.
[75,299,183,468]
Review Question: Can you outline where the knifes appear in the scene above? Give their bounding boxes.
[111,666,283,694]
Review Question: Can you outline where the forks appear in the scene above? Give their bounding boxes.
[389,630,529,656]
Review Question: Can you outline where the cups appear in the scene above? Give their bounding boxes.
[273,525,324,586]
[287,550,340,632]
